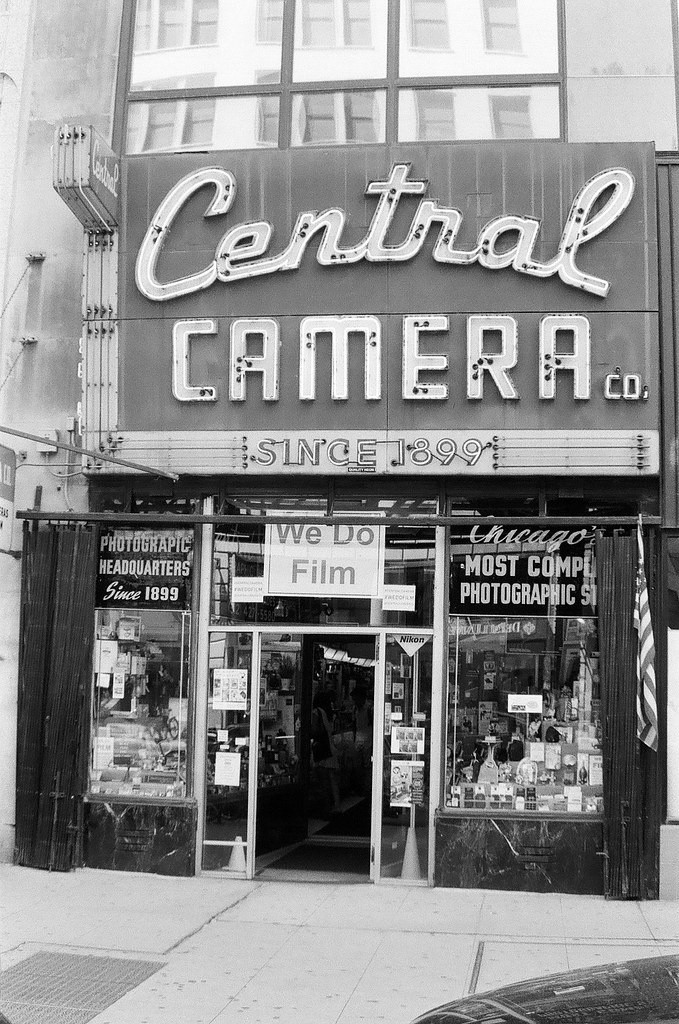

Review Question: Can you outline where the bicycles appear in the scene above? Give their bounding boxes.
[158,708,180,740]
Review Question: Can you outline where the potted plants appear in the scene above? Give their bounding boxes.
[274,654,296,691]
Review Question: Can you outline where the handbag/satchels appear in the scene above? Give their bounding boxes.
[312,708,332,762]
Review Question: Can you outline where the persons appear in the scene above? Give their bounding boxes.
[308,690,342,815]
[349,686,374,792]
[324,681,338,710]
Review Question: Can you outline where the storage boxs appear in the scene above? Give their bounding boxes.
[96,619,148,677]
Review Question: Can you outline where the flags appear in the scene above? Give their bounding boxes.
[632,516,659,752]
